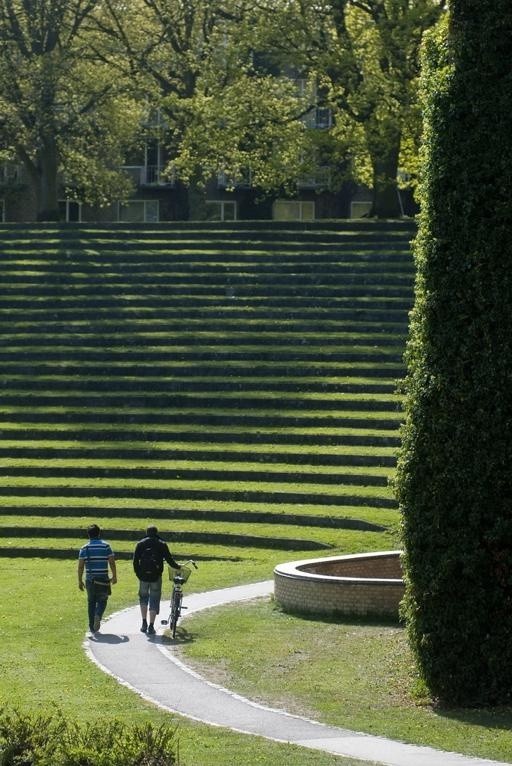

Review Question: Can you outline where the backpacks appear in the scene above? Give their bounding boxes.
[133,540,164,581]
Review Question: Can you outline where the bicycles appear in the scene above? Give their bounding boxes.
[161,551,201,640]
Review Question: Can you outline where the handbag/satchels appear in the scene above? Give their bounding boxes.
[90,576,112,597]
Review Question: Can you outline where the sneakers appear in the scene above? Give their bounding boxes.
[140,625,157,635]
[92,614,101,632]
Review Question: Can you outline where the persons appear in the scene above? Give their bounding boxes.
[77,524,119,636]
[133,524,183,636]
